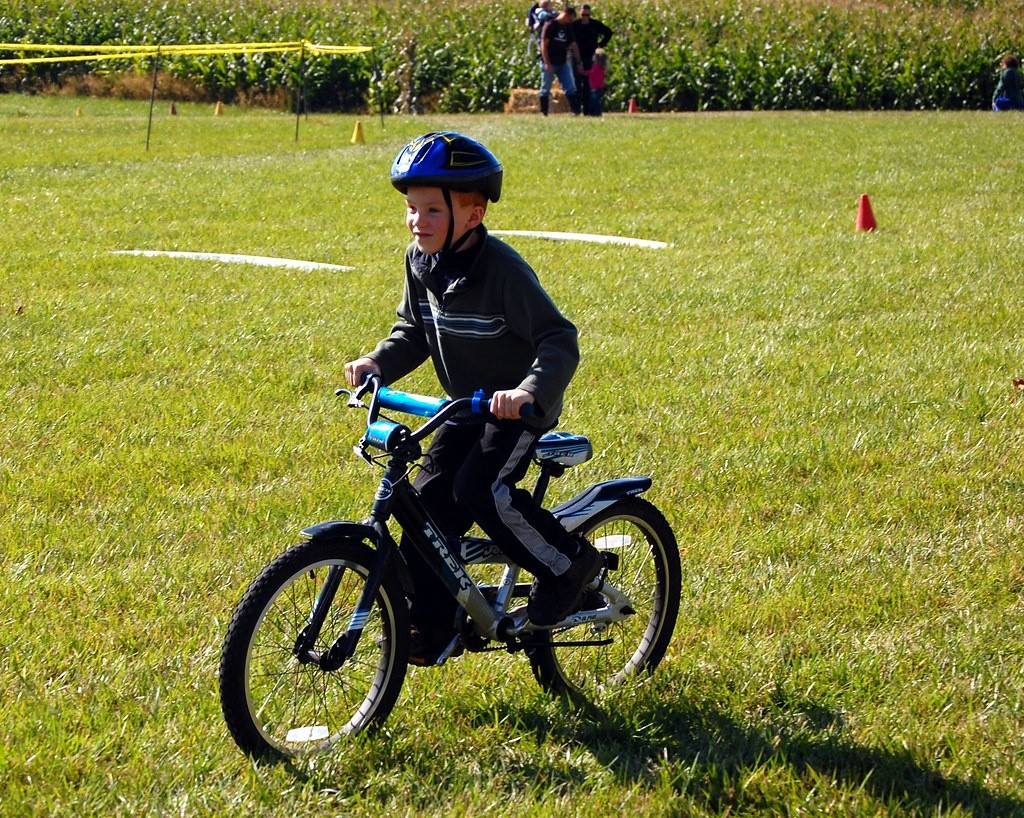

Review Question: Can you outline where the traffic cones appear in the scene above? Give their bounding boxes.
[75,107,83,118]
[628,98,638,114]
[855,194,878,234]
[351,121,366,145]
[214,101,223,116]
[169,102,177,115]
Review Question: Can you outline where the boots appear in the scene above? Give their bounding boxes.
[567,93,580,114]
[539,96,549,115]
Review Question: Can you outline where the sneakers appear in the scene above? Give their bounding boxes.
[526,533,605,627]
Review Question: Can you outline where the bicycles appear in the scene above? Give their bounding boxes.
[219,370,682,767]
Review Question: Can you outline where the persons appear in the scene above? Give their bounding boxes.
[341,132,605,657]
[992,55,1024,111]
[526,0,614,116]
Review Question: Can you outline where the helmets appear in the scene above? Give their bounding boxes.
[390,132,503,203]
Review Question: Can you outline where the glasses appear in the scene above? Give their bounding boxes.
[581,13,590,16]
